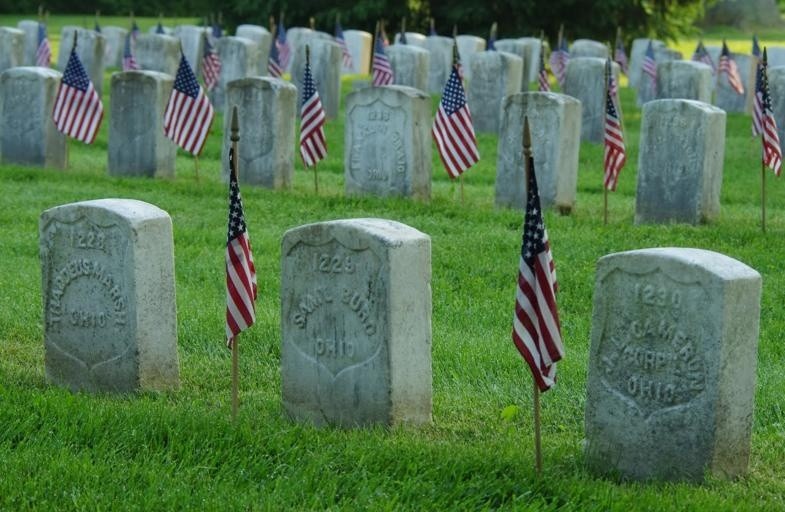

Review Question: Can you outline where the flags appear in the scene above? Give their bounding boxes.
[221,146,260,350]
[510,147,566,393]
